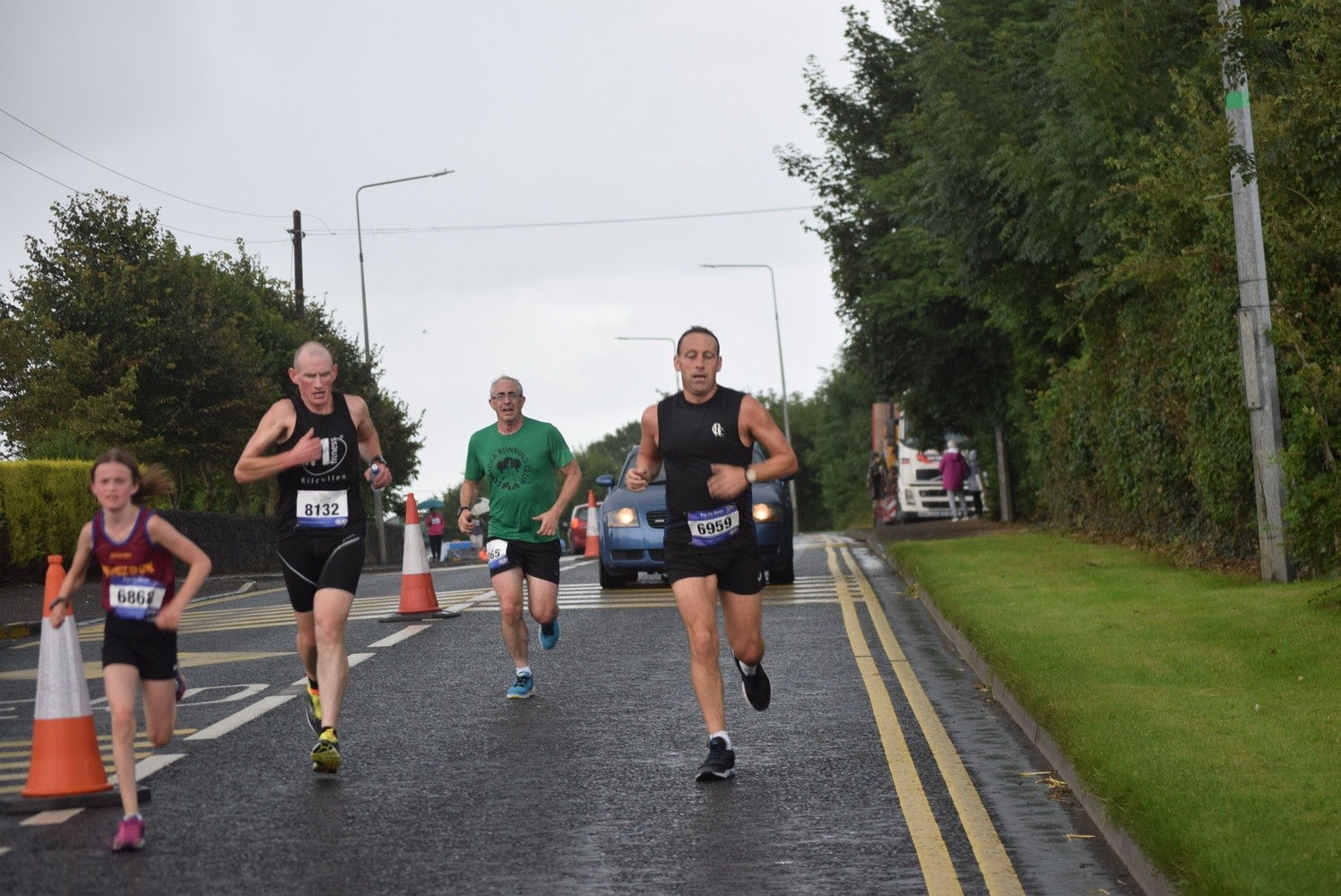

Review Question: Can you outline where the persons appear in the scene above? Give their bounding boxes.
[626,325,799,782]
[458,374,583,698]
[48,450,212,852]
[233,340,392,775]
[967,449,989,519]
[468,516,486,551]
[939,440,969,521]
[866,452,888,526]
[425,508,444,562]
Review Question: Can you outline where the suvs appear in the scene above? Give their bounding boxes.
[595,443,798,588]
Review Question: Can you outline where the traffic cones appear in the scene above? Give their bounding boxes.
[378,493,461,623]
[575,489,601,562]
[0,552,152,814]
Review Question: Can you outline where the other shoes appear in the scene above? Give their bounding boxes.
[961,516,970,521]
[952,518,959,522]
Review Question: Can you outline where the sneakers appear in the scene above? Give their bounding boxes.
[310,728,343,774]
[731,647,770,712]
[539,618,559,650]
[695,736,735,782]
[175,659,185,702]
[112,815,145,851]
[305,679,322,736]
[506,670,535,698]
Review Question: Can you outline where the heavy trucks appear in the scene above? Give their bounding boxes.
[871,387,992,523]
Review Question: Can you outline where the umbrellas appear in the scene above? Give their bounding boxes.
[471,497,490,516]
[417,499,447,509]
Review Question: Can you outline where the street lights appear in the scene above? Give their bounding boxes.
[355,168,457,366]
[700,263,799,536]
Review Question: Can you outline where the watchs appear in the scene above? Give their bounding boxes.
[50,597,69,611]
[457,507,471,517]
[370,455,387,465]
[744,465,757,484]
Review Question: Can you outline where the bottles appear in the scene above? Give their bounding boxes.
[371,464,385,491]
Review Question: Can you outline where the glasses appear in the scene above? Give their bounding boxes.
[492,393,522,399]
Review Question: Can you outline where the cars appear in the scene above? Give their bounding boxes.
[563,501,607,555]
